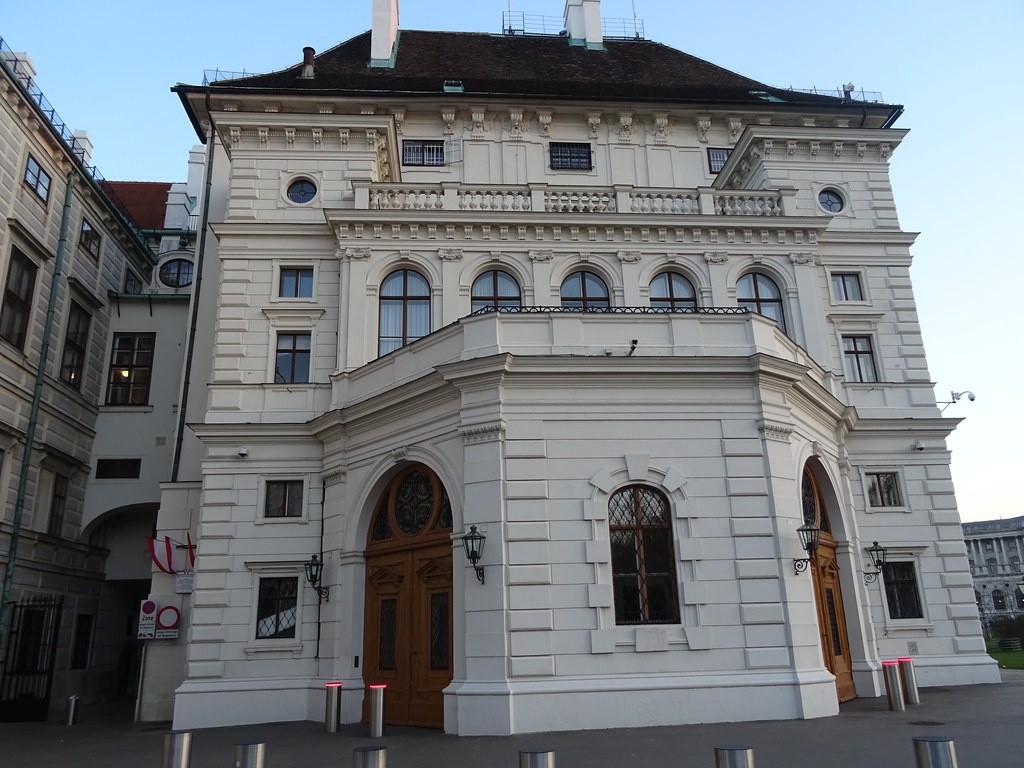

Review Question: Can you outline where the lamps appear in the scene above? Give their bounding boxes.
[460,525,487,584]
[304,554,330,602]
[793,518,821,575]
[442,80,464,94]
[863,541,887,585]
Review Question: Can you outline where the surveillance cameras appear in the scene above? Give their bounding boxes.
[968,393,975,402]
[239,449,248,457]
[916,442,925,450]
